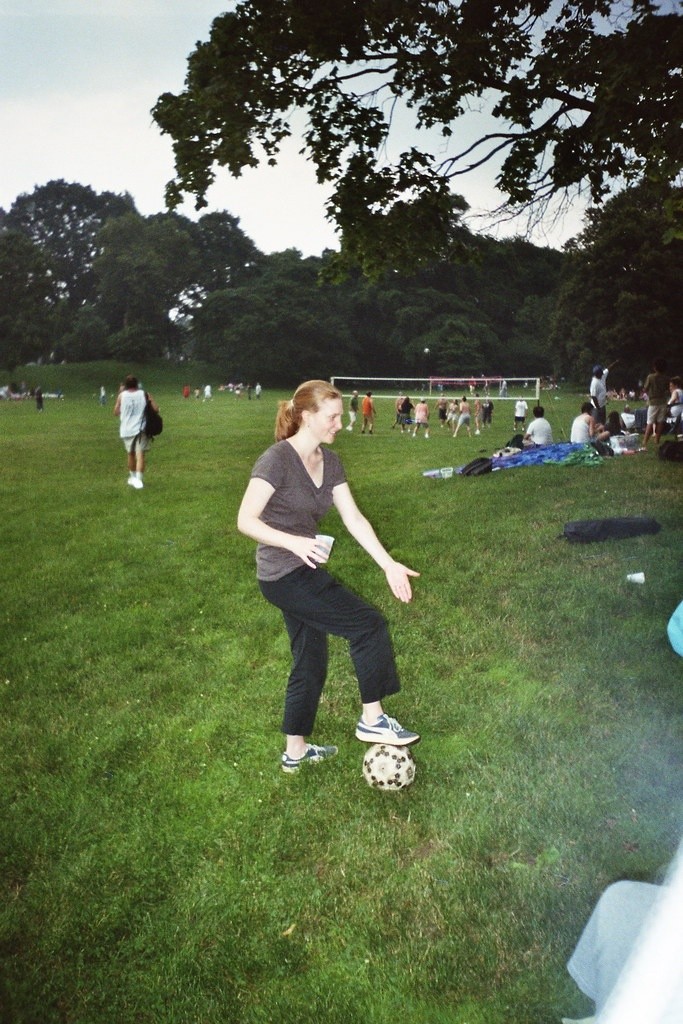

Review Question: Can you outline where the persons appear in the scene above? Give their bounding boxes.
[230,382,262,400]
[101,386,106,406]
[434,393,494,437]
[184,384,214,402]
[514,395,528,431]
[392,396,430,438]
[114,375,159,488]
[643,359,670,444]
[590,360,619,424]
[610,388,648,401]
[523,407,553,447]
[572,402,621,442]
[35,385,43,411]
[469,381,476,395]
[484,381,491,395]
[237,380,422,773]
[361,391,376,434]
[499,381,507,397]
[666,376,683,423]
[346,390,359,431]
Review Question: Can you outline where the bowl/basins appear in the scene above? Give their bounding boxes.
[441,468,453,478]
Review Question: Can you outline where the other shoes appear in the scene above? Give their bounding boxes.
[425,432,430,438]
[412,433,416,437]
[128,477,144,488]
[474,431,480,434]
[369,430,372,433]
[346,425,353,431]
[362,431,365,433]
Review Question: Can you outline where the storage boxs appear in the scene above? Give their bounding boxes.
[609,433,639,453]
[441,468,453,478]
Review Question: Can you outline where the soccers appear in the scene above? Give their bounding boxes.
[363,743,416,790]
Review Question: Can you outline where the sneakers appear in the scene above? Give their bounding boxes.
[282,743,339,773]
[356,713,420,746]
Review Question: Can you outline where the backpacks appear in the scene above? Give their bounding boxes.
[143,392,163,438]
[462,457,493,476]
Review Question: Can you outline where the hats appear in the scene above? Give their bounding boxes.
[592,365,603,375]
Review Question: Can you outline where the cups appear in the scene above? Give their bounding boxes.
[626,572,645,584]
[315,534,335,564]
